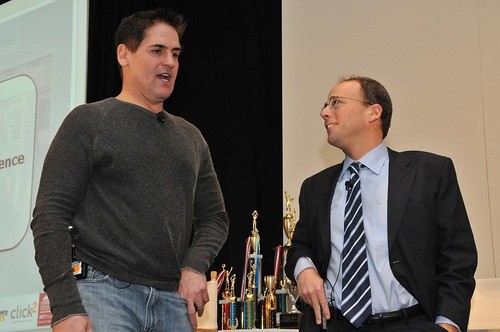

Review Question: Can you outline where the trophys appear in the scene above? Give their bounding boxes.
[217,191,301,330]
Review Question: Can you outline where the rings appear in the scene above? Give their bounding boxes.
[196,302,204,310]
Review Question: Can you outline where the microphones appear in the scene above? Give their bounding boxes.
[156,112,165,123]
[345,181,352,190]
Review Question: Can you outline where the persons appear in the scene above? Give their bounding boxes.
[284,75,478,332]
[30,8,229,332]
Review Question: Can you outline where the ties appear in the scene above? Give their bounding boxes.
[341,163,372,328]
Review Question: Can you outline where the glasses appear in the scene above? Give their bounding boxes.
[321,96,373,109]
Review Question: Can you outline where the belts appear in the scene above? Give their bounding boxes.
[304,304,425,327]
